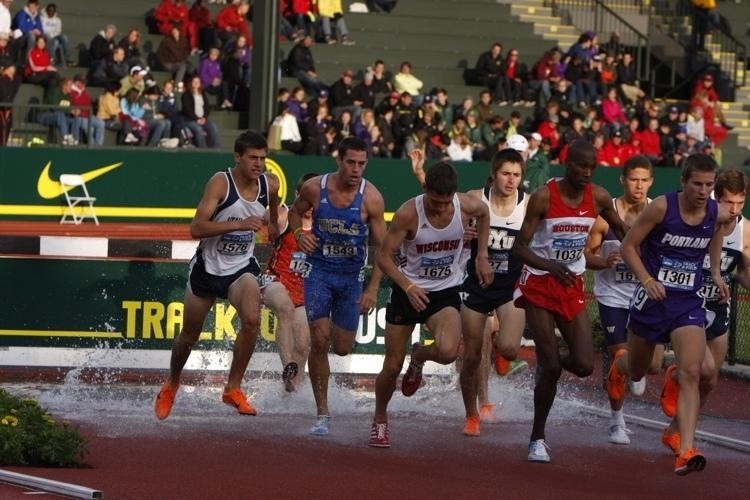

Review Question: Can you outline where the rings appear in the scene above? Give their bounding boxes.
[564,271,569,277]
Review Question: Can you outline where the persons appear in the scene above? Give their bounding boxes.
[0,0,253,149]
[258,173,320,396]
[407,147,527,425]
[369,162,496,448]
[513,140,641,463]
[584,155,663,444]
[660,171,750,458]
[606,153,730,477]
[287,137,387,436]
[460,149,532,436]
[154,132,278,421]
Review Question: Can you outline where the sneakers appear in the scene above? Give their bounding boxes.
[660,365,680,416]
[662,428,680,453]
[463,415,481,436]
[368,422,391,447]
[155,378,180,420]
[402,342,424,396]
[629,376,646,396]
[496,352,510,375]
[607,422,631,444]
[310,414,332,435]
[607,349,626,399]
[516,337,537,369]
[479,402,496,424]
[342,37,355,45]
[223,387,256,415]
[327,38,336,44]
[528,438,550,462]
[721,120,734,129]
[282,362,298,392]
[674,448,706,476]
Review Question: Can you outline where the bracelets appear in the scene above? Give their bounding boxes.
[295,233,303,244]
[404,282,418,295]
[643,277,654,287]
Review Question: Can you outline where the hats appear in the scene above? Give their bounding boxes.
[430,135,441,146]
[547,114,559,124]
[676,126,687,133]
[74,75,88,82]
[343,69,353,77]
[364,73,373,80]
[424,94,432,104]
[390,91,399,98]
[613,130,622,137]
[704,74,713,80]
[667,106,678,114]
[317,89,328,97]
[505,135,529,152]
[531,132,542,141]
[130,64,147,76]
